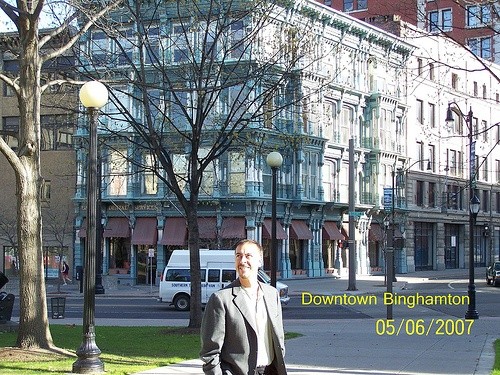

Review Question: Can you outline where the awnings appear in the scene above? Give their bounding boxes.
[322,221,346,241]
[368,224,383,242]
[198,218,217,240]
[289,221,315,239]
[221,217,247,238]
[103,218,131,238]
[161,218,188,245]
[132,217,157,246]
[262,219,288,239]
[78,219,87,238]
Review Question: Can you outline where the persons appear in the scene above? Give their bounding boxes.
[200,240,287,375]
[61,261,73,286]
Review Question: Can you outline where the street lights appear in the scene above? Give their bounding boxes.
[391,164,401,283]
[266,151,284,287]
[446,100,481,320]
[73,81,109,372]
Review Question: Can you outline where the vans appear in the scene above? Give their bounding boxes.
[158,250,291,309]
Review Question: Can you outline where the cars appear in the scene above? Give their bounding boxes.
[486,263,500,286]
